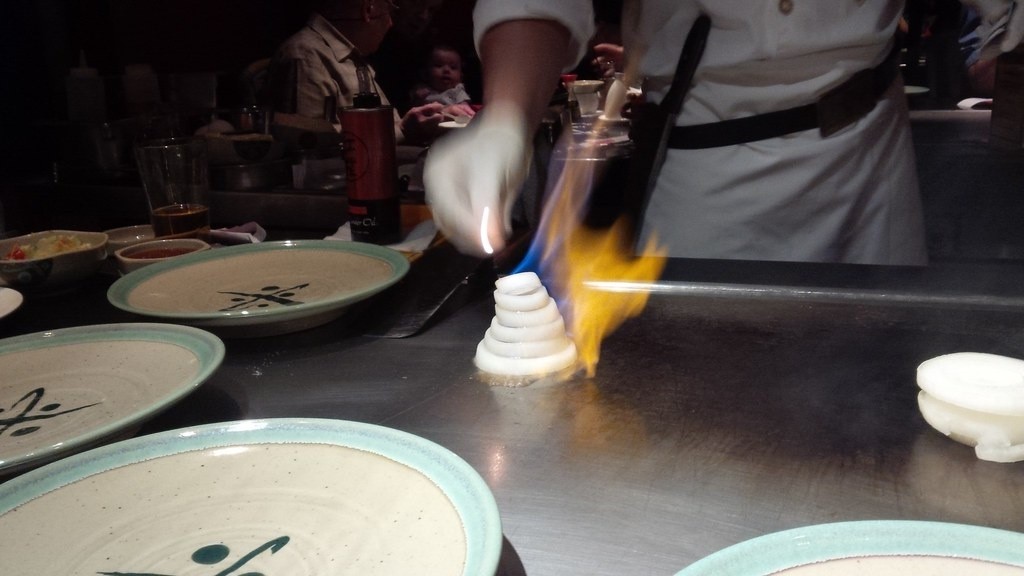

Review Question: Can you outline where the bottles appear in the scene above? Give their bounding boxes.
[605,72,626,119]
[290,145,346,193]
[338,48,402,245]
[560,74,582,127]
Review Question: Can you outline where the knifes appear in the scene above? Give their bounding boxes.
[362,258,485,338]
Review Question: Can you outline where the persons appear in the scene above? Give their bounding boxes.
[240,0,475,147]
[404,39,469,104]
[425,0,1024,264]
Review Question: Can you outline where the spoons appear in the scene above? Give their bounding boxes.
[442,111,468,123]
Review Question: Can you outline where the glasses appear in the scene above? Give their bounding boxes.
[387,0,399,17]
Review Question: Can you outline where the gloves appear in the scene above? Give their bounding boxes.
[423,100,533,258]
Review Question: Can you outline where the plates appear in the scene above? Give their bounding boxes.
[107,226,157,247]
[671,520,1024,576]
[0,288,22,321]
[0,416,504,576]
[438,120,466,128]
[0,323,228,474]
[599,114,630,124]
[562,80,605,94]
[904,85,930,94]
[106,241,412,336]
[957,97,993,109]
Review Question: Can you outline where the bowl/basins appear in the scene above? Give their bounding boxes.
[117,240,212,278]
[0,230,108,289]
[216,160,273,194]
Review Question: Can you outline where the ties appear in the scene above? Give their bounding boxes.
[350,51,370,96]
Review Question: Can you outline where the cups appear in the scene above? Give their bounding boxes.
[132,136,210,240]
[238,104,266,134]
